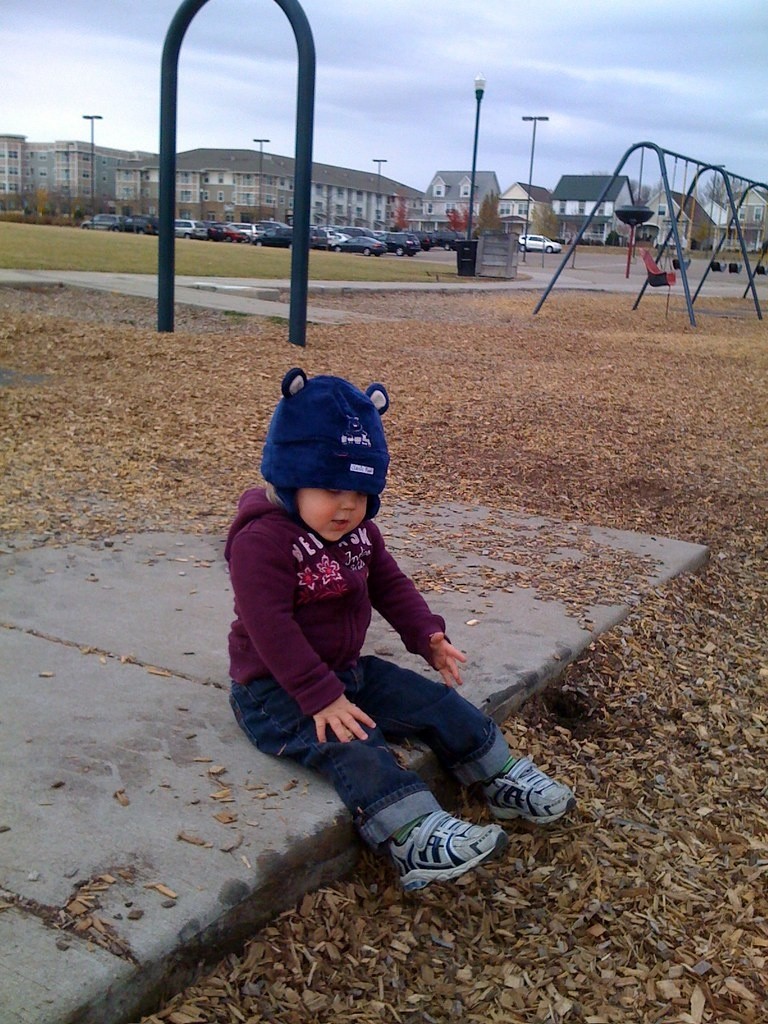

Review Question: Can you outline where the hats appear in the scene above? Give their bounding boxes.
[261,367,390,523]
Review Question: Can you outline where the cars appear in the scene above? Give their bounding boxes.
[519,234,562,253]
[80,213,124,231]
[121,213,464,257]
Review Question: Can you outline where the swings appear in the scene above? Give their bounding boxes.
[673,159,700,270]
[710,170,768,275]
[637,147,678,287]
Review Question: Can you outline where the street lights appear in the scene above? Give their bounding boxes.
[254,139,270,220]
[521,116,549,260]
[82,114,104,208]
[454,72,486,279]
[373,159,388,231]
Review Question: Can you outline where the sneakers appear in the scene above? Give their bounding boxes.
[390,809,507,890]
[480,756,577,824]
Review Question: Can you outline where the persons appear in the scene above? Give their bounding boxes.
[225,370,576,891]
